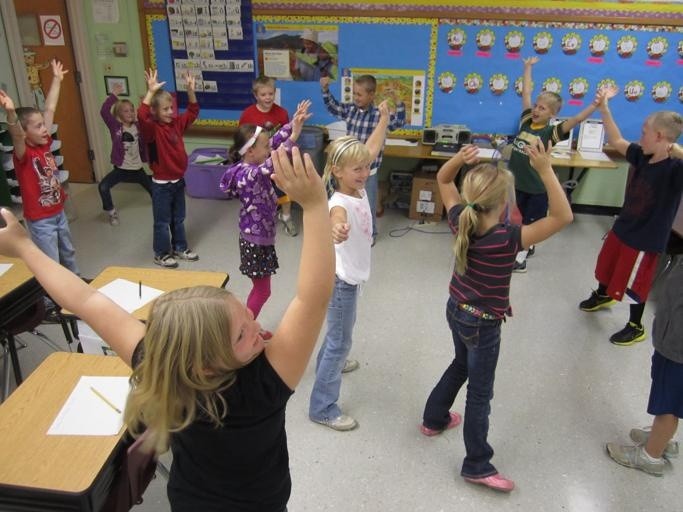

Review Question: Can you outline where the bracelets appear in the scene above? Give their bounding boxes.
[593,102,600,108]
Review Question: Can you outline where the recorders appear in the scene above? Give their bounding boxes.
[422,125,471,146]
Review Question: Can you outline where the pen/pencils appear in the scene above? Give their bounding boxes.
[138,281,142,302]
[89,385,121,414]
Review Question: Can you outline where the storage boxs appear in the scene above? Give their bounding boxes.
[409,174,443,222]
[184,148,232,200]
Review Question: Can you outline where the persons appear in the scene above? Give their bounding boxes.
[509,54,619,273]
[219,74,387,340]
[419,138,575,491]
[309,99,391,432]
[0,145,337,512]
[289,30,336,80]
[98,67,200,268]
[578,90,683,344]
[0,59,93,317]
[606,253,682,477]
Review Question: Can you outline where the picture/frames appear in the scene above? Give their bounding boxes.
[105,76,130,96]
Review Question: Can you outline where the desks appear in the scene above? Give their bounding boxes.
[383,135,618,206]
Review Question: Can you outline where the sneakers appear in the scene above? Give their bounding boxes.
[630,429,678,457]
[110,209,118,225]
[609,322,645,346]
[172,249,199,261]
[606,443,665,476]
[464,473,514,492]
[41,309,59,324]
[278,212,298,237]
[341,360,358,372]
[310,414,357,431]
[153,253,178,267]
[580,290,615,311]
[511,245,535,273]
[422,411,461,436]
[259,330,271,342]
